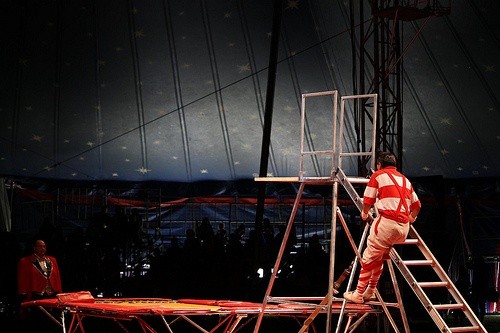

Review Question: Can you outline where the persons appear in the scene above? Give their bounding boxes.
[19,239,63,313]
[344,153,421,303]
[85,204,320,285]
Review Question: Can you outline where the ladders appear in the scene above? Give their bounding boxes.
[337,168,488,333]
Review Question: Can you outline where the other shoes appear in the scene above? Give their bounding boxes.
[362,291,376,300]
[343,291,364,303]
